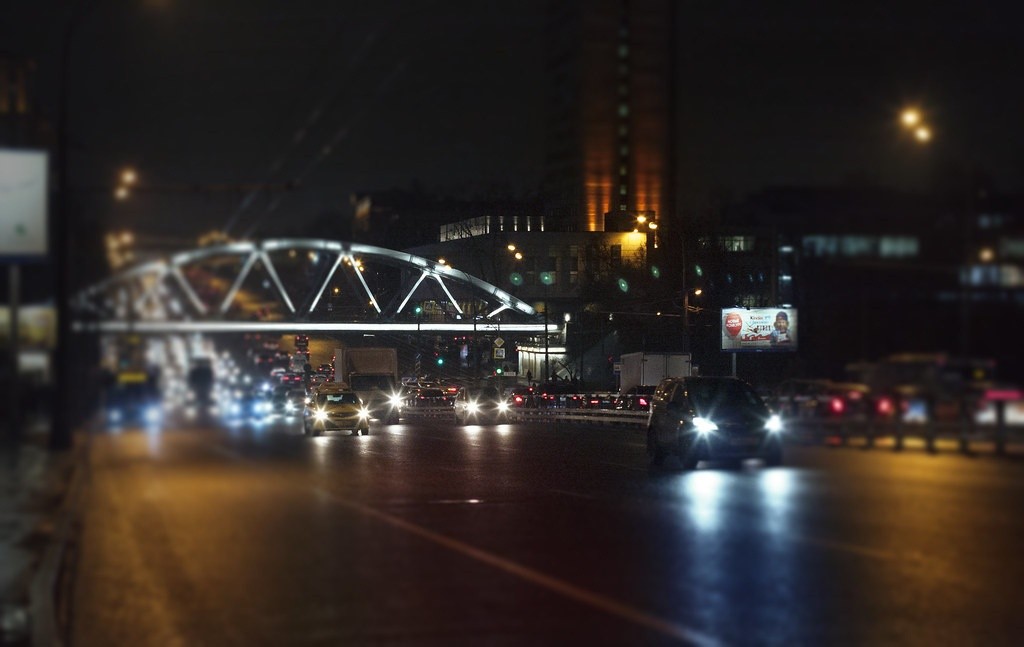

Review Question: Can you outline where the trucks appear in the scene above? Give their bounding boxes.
[335,347,399,425]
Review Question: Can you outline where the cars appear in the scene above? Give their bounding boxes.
[645,375,780,469]
[400,377,649,410]
[246,335,334,387]
[303,389,368,435]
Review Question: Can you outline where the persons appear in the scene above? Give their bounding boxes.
[770,312,790,344]
